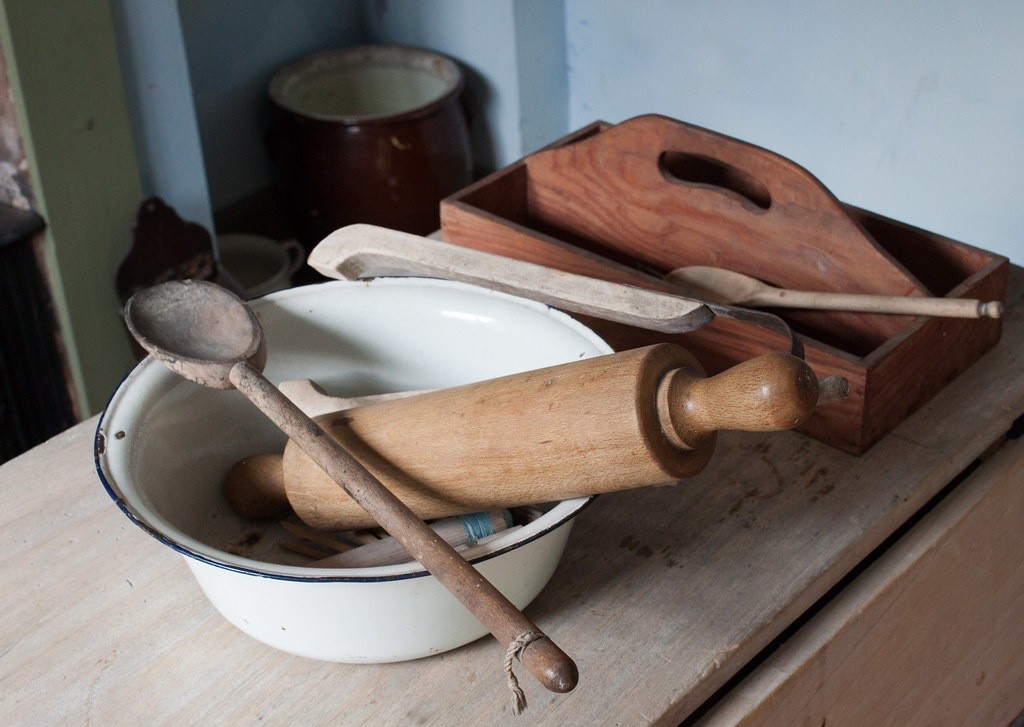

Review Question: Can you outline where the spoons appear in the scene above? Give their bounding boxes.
[121,276,579,713]
[668,265,1001,323]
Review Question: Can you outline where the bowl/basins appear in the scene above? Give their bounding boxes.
[92,270,620,666]
